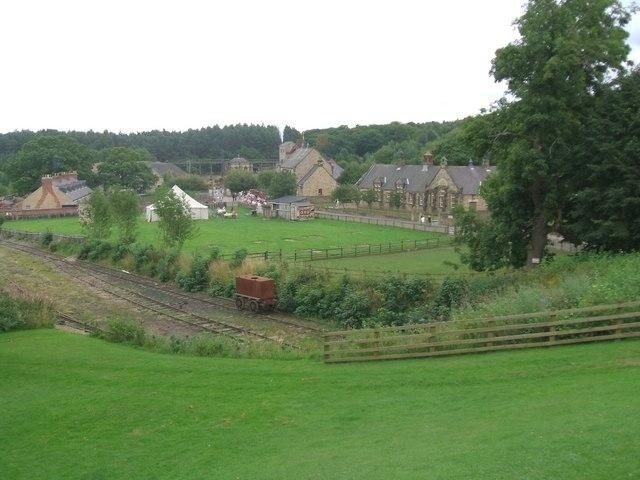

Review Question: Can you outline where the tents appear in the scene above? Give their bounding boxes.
[145,184,209,223]
